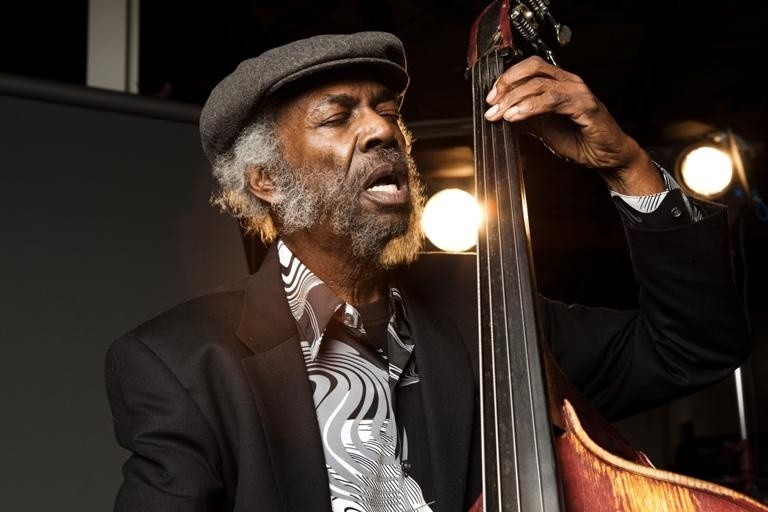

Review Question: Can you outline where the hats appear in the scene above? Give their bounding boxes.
[199,31,410,163]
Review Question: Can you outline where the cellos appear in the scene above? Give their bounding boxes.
[469,1,767,512]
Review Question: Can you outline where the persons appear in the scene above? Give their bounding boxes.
[105,31,750,512]
